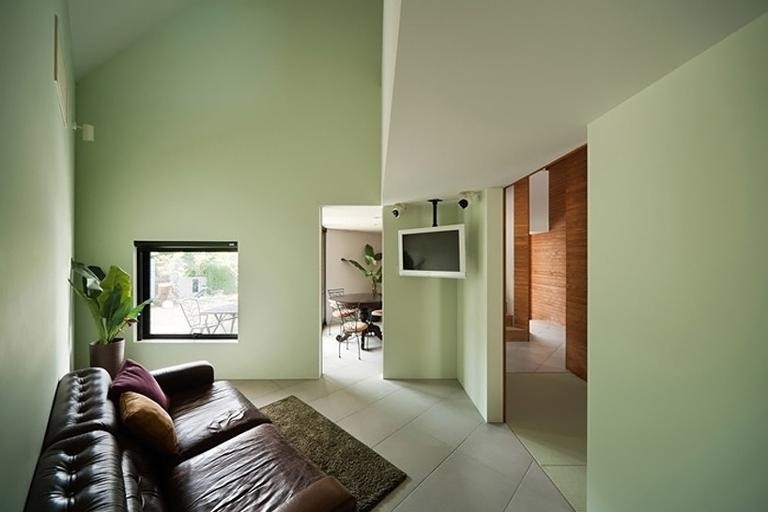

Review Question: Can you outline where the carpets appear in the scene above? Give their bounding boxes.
[255,394,407,512]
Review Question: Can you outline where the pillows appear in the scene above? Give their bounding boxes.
[107,359,174,413]
[118,391,178,453]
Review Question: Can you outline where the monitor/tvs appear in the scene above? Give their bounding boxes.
[398,223,466,280]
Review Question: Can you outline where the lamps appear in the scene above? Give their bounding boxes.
[70,120,94,143]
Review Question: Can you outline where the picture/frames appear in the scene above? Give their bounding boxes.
[54,13,69,127]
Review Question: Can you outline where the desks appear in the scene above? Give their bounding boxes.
[328,292,383,350]
[200,303,239,333]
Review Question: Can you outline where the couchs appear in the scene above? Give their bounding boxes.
[24,360,358,512]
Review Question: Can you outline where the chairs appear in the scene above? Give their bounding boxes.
[180,298,216,336]
[328,287,359,336]
[371,310,382,350]
[336,300,368,360]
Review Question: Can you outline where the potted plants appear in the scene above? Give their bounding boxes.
[67,257,154,379]
[341,244,383,296]
